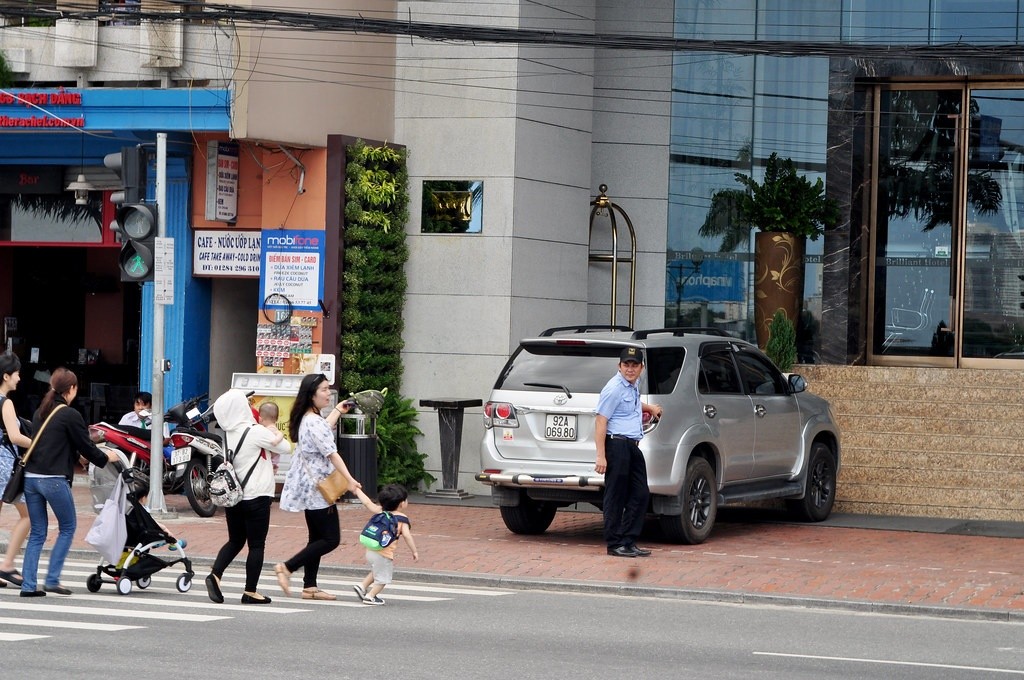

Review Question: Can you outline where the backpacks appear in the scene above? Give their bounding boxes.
[359,511,410,550]
[209,424,262,507]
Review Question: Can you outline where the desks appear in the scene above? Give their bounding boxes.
[419,396,483,500]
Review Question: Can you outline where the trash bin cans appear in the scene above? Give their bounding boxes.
[338,410,379,504]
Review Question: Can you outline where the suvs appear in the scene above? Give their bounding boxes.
[474,322,843,547]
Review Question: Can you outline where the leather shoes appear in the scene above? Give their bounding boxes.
[607,546,651,556]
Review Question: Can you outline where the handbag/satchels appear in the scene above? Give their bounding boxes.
[319,468,350,504]
[2,458,24,504]
[85,473,127,566]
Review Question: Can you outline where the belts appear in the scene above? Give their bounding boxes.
[606,435,639,446]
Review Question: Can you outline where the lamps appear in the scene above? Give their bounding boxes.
[65,132,95,207]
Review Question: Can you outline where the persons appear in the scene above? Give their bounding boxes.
[0,352,32,588]
[129,473,187,551]
[273,373,362,600]
[20,368,121,597]
[258,402,283,476]
[118,392,171,503]
[205,388,292,605]
[594,348,663,558]
[353,484,419,605]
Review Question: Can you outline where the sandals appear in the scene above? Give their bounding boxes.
[272,563,293,597]
[303,590,337,600]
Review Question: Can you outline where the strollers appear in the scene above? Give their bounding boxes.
[88,444,195,595]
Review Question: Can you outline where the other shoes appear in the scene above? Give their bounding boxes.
[0,570,22,586]
[0,580,7,587]
[44,586,72,595]
[152,535,166,548]
[169,539,187,551]
[241,592,271,604]
[20,590,46,597]
[205,574,224,603]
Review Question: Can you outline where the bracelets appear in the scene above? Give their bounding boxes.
[334,407,344,416]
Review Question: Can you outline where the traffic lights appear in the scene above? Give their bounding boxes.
[118,203,156,281]
[102,153,126,231]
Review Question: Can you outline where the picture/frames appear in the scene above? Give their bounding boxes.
[422,179,484,233]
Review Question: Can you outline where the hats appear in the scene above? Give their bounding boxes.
[620,347,643,363]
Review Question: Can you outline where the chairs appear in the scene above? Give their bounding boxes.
[880,288,937,353]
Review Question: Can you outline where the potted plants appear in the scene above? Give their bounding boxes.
[733,150,846,357]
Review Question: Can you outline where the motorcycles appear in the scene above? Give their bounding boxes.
[87,392,211,516]
[170,391,257,518]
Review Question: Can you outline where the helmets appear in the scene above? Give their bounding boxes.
[351,387,389,414]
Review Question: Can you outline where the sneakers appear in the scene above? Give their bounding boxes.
[363,594,385,604]
[354,585,367,600]
